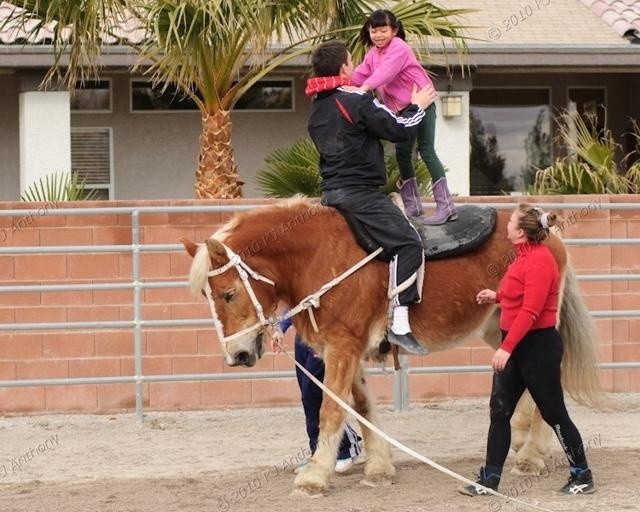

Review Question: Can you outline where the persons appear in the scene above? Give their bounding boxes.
[305,41,437,356]
[271,306,362,474]
[351,10,459,226]
[457,203,596,497]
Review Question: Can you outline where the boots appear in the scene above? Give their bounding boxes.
[396,177,425,217]
[423,176,458,225]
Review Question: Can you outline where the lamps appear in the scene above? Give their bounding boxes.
[442,83,462,118]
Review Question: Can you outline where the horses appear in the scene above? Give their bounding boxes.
[177,192,622,499]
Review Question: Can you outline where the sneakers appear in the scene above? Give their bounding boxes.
[457,465,501,496]
[294,461,309,473]
[334,446,367,473]
[555,467,596,496]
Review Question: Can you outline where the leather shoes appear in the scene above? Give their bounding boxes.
[388,328,429,355]
